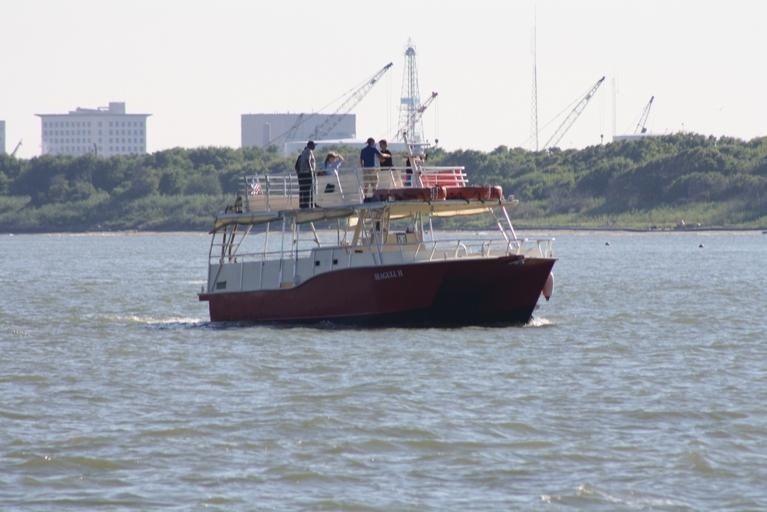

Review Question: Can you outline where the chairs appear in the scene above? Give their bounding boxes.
[377,169,403,189]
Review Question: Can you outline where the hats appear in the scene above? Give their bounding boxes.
[327,152,336,159]
[365,138,387,144]
[307,140,318,146]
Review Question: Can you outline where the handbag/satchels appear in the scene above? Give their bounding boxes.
[324,184,336,193]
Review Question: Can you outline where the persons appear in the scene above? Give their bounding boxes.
[378,139,396,170]
[319,152,344,193]
[405,156,413,186]
[295,141,320,209]
[360,138,391,199]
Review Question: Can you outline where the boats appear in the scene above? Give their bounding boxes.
[198,132,558,328]
